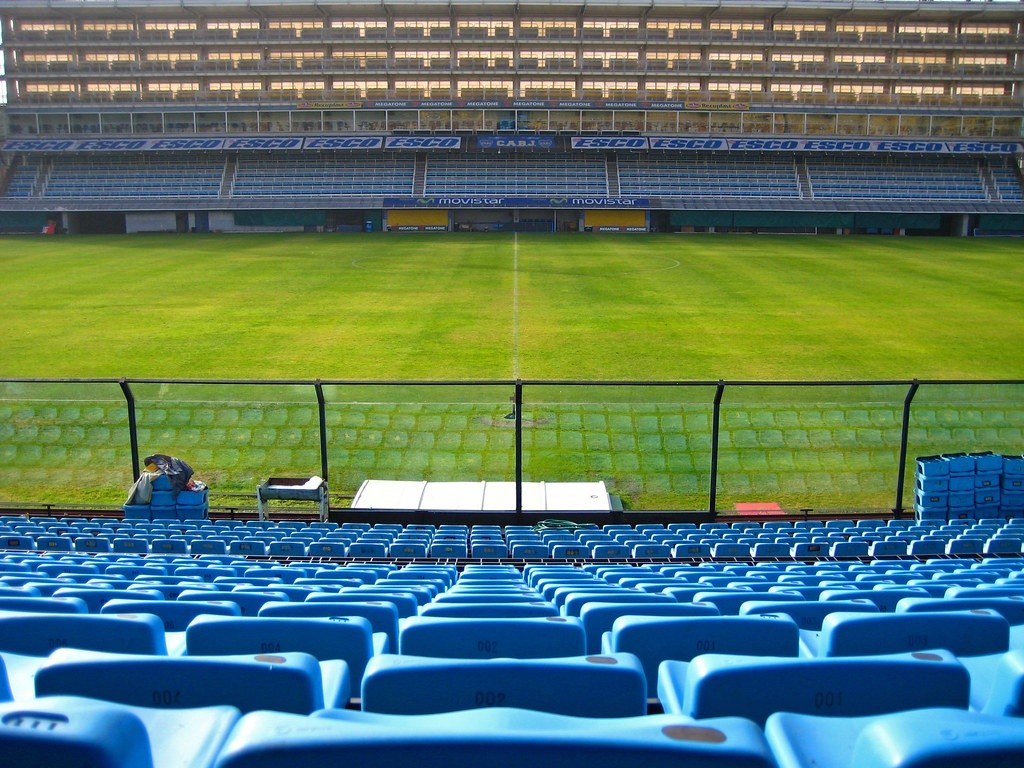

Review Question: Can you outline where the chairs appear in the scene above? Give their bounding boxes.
[0,516,1024,768]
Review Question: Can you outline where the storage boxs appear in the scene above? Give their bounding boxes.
[911,452,1024,519]
[177,502,208,520]
[123,504,152,520]
[151,506,177,519]
[176,489,208,505]
[151,475,173,491]
[150,490,176,506]
